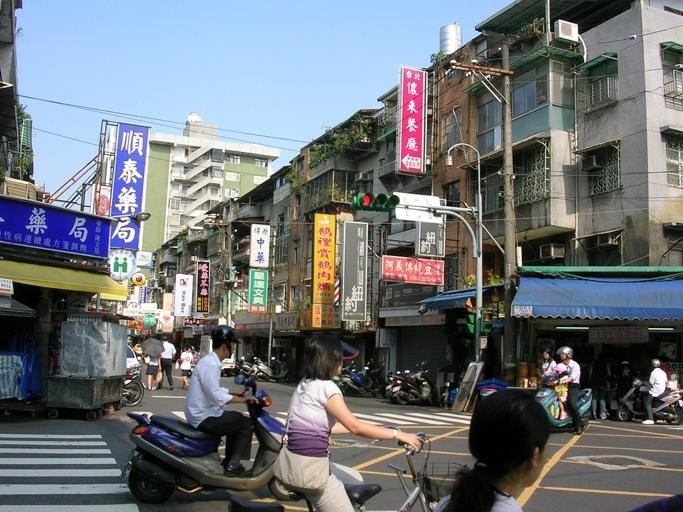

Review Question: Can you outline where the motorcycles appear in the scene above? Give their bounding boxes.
[119,375,303,504]
[616,379,683,425]
[535,369,593,431]
[121,367,147,407]
[239,355,291,384]
[338,358,436,405]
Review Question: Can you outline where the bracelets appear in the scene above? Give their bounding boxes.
[391,428,397,441]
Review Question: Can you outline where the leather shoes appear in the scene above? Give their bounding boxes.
[576,425,582,435]
[223,458,255,477]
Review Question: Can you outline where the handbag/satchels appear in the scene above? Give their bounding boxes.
[269,438,331,493]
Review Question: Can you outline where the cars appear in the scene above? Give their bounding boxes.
[127,344,140,369]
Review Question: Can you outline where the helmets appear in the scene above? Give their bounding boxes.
[554,362,568,375]
[651,358,661,368]
[556,345,574,359]
[209,324,242,346]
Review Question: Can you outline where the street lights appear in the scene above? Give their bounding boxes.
[111,212,151,221]
[445,143,483,362]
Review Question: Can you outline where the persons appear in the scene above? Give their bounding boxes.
[284,332,425,512]
[143,354,162,392]
[433,388,552,512]
[640,357,668,425]
[176,343,193,390]
[184,323,261,479]
[552,363,570,422]
[555,346,585,435]
[539,346,557,378]
[156,336,177,391]
[587,351,613,421]
[614,355,638,406]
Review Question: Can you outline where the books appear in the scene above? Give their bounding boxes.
[139,337,166,357]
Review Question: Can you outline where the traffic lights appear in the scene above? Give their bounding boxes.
[354,191,399,210]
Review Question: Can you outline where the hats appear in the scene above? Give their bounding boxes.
[337,338,361,361]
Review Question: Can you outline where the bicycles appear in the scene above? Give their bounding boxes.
[228,425,464,512]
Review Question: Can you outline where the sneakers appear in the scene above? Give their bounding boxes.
[642,419,655,425]
[554,411,567,420]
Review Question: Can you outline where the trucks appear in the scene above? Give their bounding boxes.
[196,335,235,377]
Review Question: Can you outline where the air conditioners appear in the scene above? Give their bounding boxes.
[597,234,618,245]
[554,19,578,44]
[355,172,369,183]
[582,155,602,172]
[540,243,565,260]
